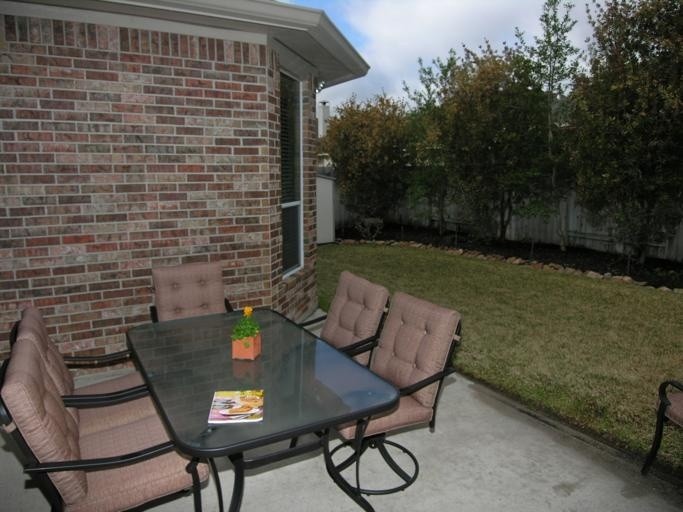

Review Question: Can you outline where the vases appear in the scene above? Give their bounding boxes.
[231,333,262,360]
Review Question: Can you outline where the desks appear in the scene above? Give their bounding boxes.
[127,306,400,511]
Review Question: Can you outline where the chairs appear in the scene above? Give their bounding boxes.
[325,290,463,495]
[1,338,210,511]
[15,306,157,439]
[298,270,392,352]
[149,260,233,324]
[639,380,682,476]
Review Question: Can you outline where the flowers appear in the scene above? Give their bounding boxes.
[230,306,262,336]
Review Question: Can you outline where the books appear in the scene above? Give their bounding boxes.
[207,389,264,425]
